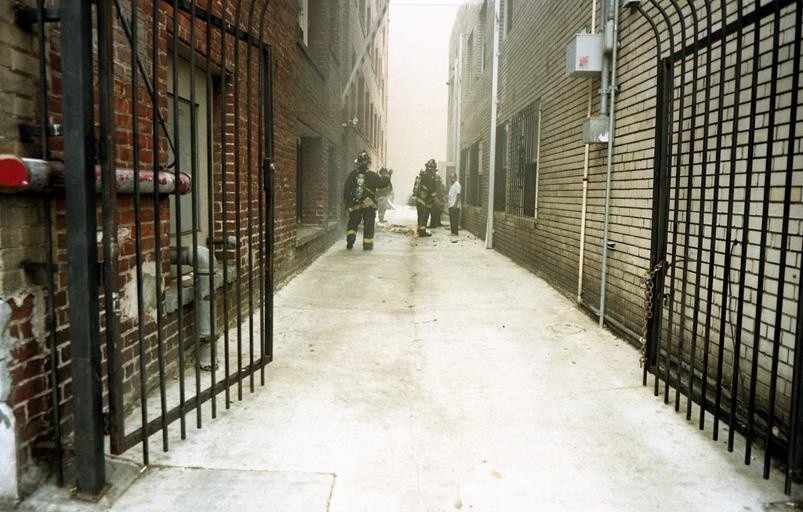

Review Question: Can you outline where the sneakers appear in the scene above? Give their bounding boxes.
[347,239,354,248]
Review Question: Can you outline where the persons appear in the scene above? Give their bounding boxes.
[447,172,461,235]
[342,150,391,250]
[426,176,444,228]
[412,158,439,237]
[374,167,392,222]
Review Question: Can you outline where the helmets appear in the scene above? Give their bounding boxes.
[354,152,371,165]
[425,159,438,172]
